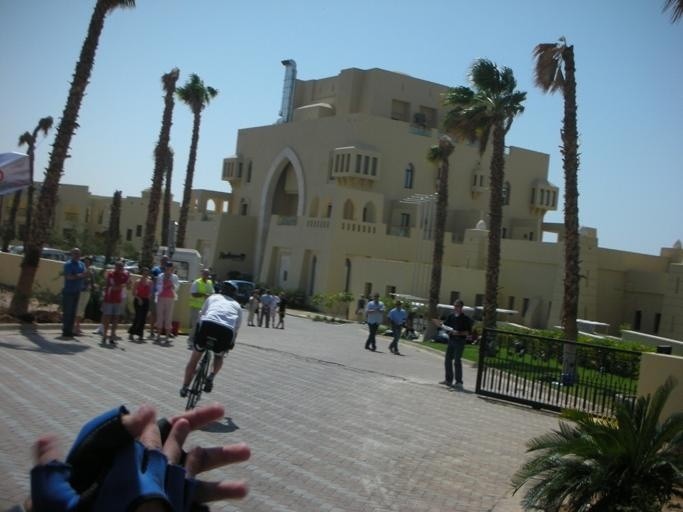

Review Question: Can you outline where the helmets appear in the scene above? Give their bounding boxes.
[223,281,239,293]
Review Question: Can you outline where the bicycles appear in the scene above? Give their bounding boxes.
[178,342,219,414]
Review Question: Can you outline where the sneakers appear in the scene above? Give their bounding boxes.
[59,327,175,347]
[178,383,189,398]
[246,323,285,330]
[364,345,400,356]
[439,380,463,389]
[203,376,214,393]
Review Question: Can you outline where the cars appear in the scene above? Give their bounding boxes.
[14,245,140,274]
[218,278,255,304]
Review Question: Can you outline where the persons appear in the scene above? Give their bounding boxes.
[248,288,287,329]
[180,280,241,398]
[434,300,473,389]
[61,246,218,348]
[28,400,252,512]
[355,294,365,315]
[387,301,406,355]
[365,293,384,351]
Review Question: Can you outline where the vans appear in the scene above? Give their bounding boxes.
[153,246,203,282]
